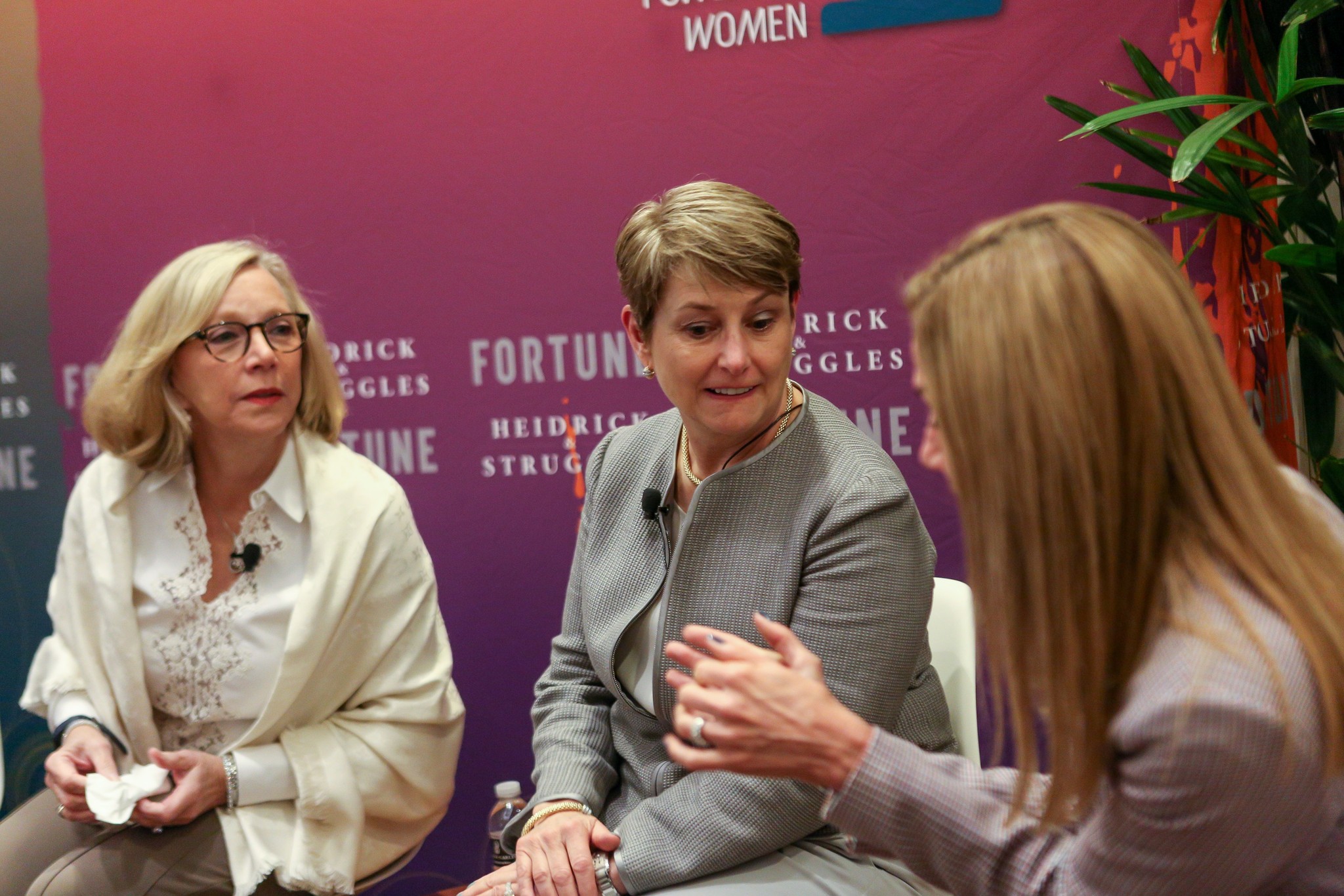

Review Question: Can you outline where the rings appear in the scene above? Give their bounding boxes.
[152,826,164,835]
[687,716,710,750]
[52,803,66,816]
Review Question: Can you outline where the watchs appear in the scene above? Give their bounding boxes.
[588,845,619,896]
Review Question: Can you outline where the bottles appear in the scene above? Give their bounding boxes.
[488,781,529,870]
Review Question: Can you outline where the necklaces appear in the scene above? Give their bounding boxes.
[681,377,793,485]
[178,470,267,556]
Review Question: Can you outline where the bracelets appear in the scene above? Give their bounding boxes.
[511,799,608,837]
[220,748,245,819]
[59,718,104,743]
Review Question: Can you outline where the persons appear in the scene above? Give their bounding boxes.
[461,177,960,896]
[663,196,1344,896]
[0,238,470,896]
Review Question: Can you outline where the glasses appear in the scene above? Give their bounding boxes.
[183,312,311,364]
